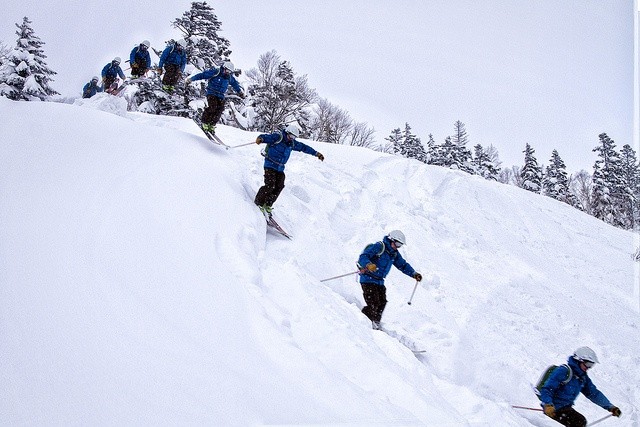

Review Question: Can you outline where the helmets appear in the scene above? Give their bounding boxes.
[388,230,405,243]
[285,125,299,137]
[223,62,234,72]
[177,39,187,47]
[573,346,597,363]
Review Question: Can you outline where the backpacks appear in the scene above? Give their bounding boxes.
[206,65,226,94]
[533,364,574,405]
[260,128,286,166]
[355,241,385,280]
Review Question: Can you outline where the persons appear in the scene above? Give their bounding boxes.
[538,346,622,427]
[129,40,152,80]
[185,61,244,137]
[82,76,104,99]
[254,123,325,219]
[157,38,187,92]
[101,57,126,94]
[355,229,423,331]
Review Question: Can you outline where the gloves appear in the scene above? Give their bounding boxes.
[255,138,262,143]
[366,263,376,272]
[609,406,621,416]
[413,272,421,281]
[315,152,324,161]
[543,404,556,418]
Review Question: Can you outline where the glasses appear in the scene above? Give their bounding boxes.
[393,239,403,248]
[225,67,232,73]
[584,360,594,368]
[288,132,297,139]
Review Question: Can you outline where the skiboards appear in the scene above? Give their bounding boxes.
[192,113,227,147]
[106,83,124,95]
[266,216,293,238]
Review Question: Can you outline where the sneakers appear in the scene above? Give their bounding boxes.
[162,85,170,90]
[208,123,215,134]
[170,85,174,91]
[201,123,209,131]
